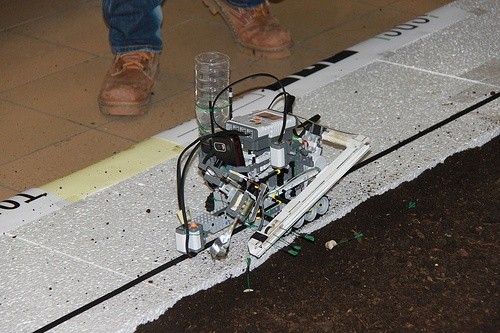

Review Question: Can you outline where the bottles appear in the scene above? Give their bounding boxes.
[195,53,230,135]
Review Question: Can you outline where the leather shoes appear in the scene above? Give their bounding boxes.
[98,50,160,116]
[202,0,294,60]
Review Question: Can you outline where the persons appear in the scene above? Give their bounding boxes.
[97,0,295,118]
